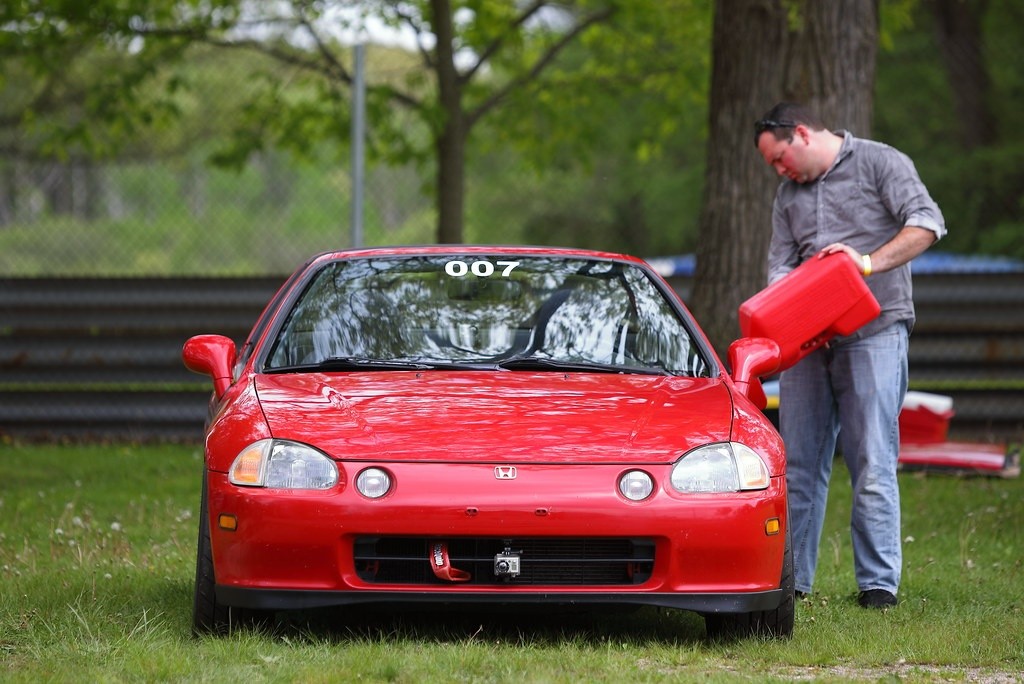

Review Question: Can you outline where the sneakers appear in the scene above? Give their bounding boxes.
[796,590,805,600]
[859,589,897,609]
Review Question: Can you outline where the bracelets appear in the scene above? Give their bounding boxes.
[861,254,872,280]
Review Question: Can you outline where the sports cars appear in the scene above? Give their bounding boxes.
[180,242,798,651]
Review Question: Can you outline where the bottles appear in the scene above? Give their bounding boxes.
[737,251,880,382]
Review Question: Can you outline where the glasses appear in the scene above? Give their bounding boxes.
[754,120,795,134]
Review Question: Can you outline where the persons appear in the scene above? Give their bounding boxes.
[753,104,950,607]
